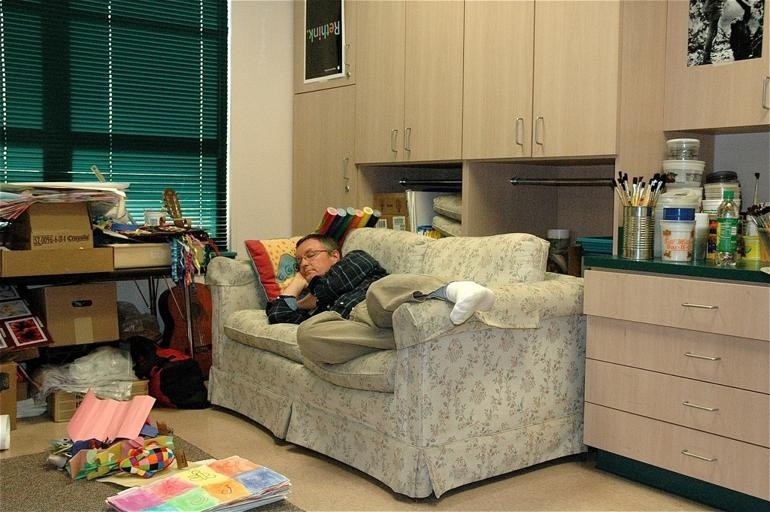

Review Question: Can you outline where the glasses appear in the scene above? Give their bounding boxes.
[296,250,329,264]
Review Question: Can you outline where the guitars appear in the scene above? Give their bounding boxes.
[157,189,213,378]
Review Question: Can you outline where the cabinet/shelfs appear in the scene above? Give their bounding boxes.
[664,0,770,137]
[358,1,463,159]
[463,0,621,159]
[294,0,357,235]
[580,254,770,512]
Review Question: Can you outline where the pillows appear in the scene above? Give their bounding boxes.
[244,233,302,305]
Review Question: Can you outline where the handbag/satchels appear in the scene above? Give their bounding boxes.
[129,334,206,408]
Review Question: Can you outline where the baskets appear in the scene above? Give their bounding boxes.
[575,236,613,253]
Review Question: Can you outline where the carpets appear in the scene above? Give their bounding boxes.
[0,430,308,511]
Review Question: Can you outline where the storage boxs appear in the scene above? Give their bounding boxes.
[43,380,150,424]
[12,204,96,252]
[0,359,17,430]
[25,281,120,347]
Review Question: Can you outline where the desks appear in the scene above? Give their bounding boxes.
[0,264,216,422]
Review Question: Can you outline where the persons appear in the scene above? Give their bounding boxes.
[266,234,495,365]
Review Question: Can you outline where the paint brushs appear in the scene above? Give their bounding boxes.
[745,205,770,228]
[753,172,760,204]
[611,171,669,207]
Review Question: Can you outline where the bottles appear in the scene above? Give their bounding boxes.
[660,220,694,262]
[714,191,741,267]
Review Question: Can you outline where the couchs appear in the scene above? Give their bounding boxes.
[205,228,585,501]
